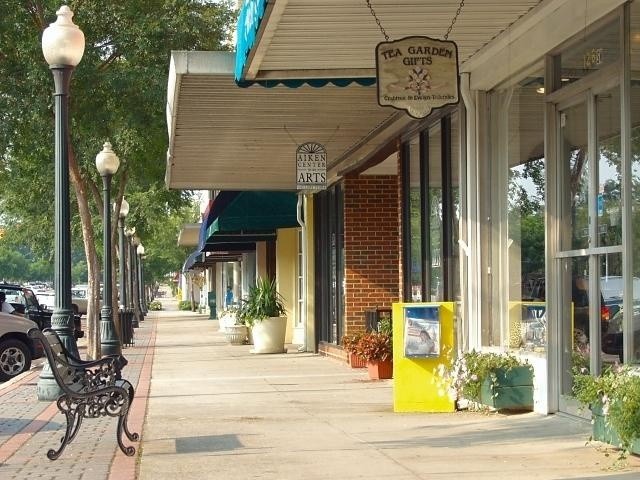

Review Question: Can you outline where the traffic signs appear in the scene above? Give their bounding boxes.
[580,225,607,238]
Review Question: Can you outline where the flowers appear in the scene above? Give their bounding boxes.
[433,348,521,401]
[565,350,640,470]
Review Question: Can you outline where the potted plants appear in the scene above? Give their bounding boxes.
[240,274,287,354]
[342,331,392,380]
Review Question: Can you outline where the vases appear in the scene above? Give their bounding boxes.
[590,400,640,456]
[225,325,247,346]
[465,365,534,410]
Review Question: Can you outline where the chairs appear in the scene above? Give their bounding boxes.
[27,326,140,460]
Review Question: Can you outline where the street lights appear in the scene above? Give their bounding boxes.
[113,194,148,344]
[35,4,86,399]
[95,141,128,380]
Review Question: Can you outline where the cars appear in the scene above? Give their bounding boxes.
[0,281,104,382]
[523,275,640,365]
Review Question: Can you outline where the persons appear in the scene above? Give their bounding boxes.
[226,285,233,309]
[417,330,435,354]
[0,291,26,319]
